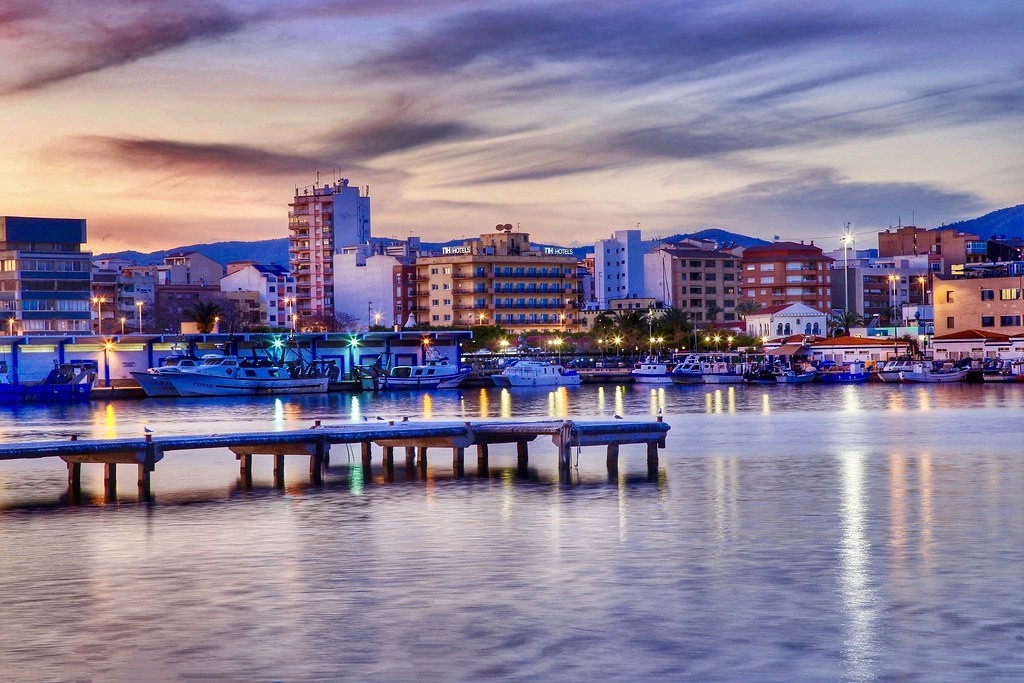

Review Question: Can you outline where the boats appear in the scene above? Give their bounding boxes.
[0,359,98,403]
[629,356,673,384]
[672,352,820,384]
[879,363,973,383]
[981,360,1024,382]
[822,361,873,385]
[340,345,476,393]
[460,361,586,388]
[129,335,335,396]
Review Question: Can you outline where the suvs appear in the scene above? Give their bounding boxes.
[568,358,590,369]
[596,358,625,368]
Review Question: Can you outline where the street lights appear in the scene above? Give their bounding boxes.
[284,296,299,334]
[548,339,562,364]
[841,236,853,314]
[137,300,143,333]
[9,319,13,334]
[706,335,734,351]
[121,317,126,334]
[920,278,928,361]
[888,274,901,361]
[92,296,104,335]
[615,337,622,357]
[501,340,509,358]
[649,336,664,356]
